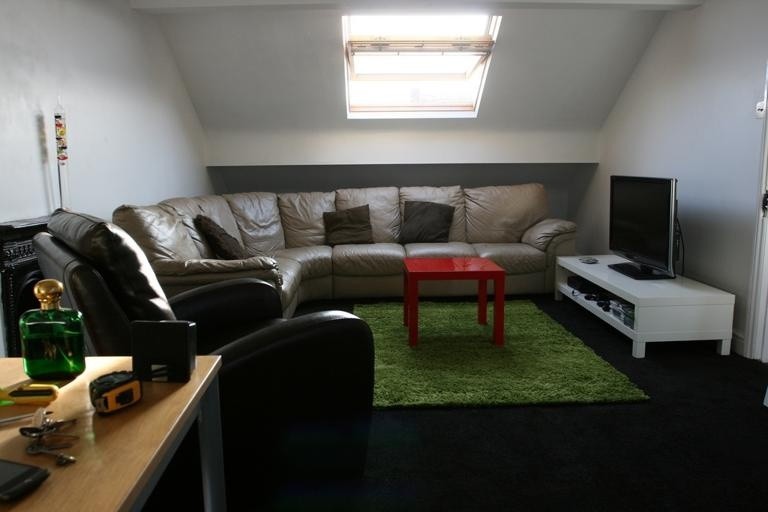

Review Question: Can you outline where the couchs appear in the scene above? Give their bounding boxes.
[113,183,577,333]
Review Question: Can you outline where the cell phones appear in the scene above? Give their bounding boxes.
[0,458,51,506]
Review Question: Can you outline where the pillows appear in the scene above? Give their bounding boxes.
[194,215,256,260]
[399,201,455,244]
[323,201,375,245]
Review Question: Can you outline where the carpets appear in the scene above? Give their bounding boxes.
[353,300,651,409]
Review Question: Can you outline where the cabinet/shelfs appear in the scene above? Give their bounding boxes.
[555,254,736,358]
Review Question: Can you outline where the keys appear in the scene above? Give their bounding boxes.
[25,444,75,466]
[41,442,72,450]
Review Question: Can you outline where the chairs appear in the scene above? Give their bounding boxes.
[32,207,375,507]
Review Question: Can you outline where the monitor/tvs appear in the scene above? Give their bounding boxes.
[609,175,677,280]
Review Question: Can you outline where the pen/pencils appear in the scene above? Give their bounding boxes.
[0,411,53,427]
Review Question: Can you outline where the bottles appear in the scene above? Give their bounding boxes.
[19,277,86,381]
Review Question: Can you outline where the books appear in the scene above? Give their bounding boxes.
[608,300,634,329]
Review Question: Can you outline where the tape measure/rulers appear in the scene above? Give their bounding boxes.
[89,371,143,416]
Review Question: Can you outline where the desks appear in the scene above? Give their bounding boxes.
[0,355,228,512]
